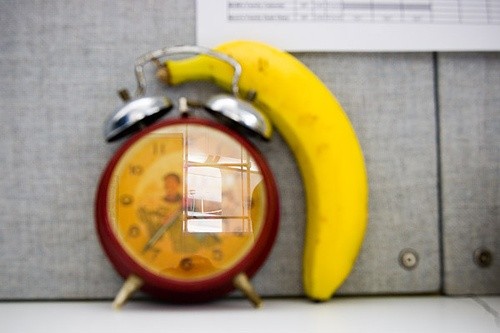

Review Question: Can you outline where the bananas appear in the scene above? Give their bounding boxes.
[157,41,369,303]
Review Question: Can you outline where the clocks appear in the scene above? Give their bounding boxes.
[93,45,280,309]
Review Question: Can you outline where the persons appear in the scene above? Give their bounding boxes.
[163,173,184,201]
